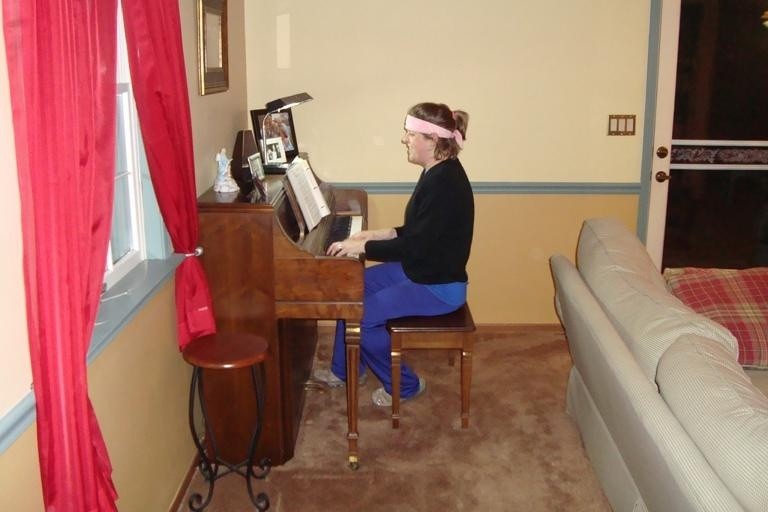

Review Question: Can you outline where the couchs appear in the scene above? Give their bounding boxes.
[550,214,767,512]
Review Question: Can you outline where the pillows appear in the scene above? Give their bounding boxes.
[663,263,767,371]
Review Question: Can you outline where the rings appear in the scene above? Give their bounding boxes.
[338,244,342,249]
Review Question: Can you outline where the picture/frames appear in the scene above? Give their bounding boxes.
[250,107,300,166]
[195,0,232,94]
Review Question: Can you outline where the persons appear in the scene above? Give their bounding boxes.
[214,148,233,184]
[259,118,290,159]
[314,102,476,407]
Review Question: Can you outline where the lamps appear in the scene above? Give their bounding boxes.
[262,92,314,177]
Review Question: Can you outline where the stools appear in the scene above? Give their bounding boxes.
[383,303,477,431]
[180,328,275,512]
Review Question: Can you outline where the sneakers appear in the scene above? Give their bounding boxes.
[309,367,368,388]
[370,375,426,407]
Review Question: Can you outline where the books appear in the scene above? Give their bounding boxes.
[286,159,332,232]
